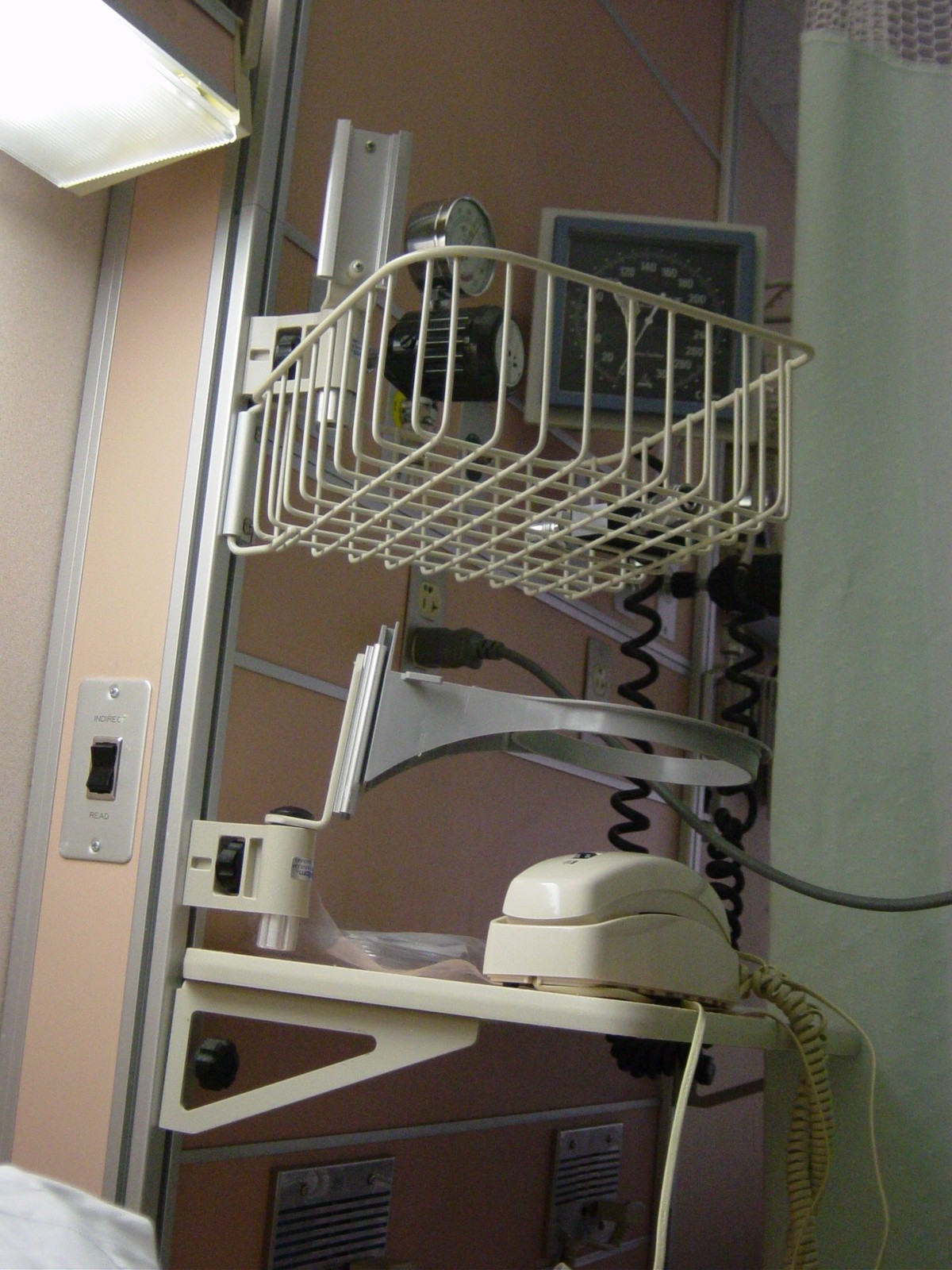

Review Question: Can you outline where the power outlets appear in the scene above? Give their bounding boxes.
[584,636,610,702]
[401,560,450,683]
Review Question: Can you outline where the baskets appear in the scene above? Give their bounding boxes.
[224,245,815,606]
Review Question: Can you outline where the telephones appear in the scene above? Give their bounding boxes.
[482,850,741,1001]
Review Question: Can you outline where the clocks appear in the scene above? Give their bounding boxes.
[524,206,766,444]
